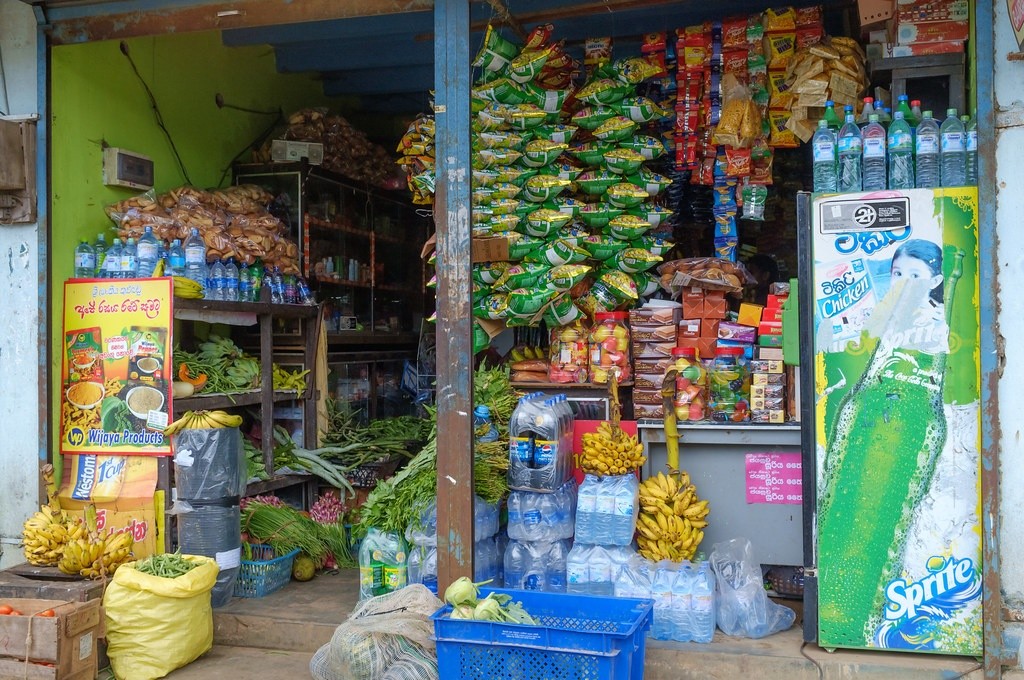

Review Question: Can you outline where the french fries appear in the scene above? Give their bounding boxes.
[63,376,122,439]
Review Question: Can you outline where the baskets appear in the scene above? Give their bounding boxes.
[427,587,659,679]
[235,539,303,598]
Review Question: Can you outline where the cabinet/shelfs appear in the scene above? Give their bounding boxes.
[155,156,438,553]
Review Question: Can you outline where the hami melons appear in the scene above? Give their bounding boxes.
[292,554,315,580]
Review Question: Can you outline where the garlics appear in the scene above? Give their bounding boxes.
[241,491,342,522]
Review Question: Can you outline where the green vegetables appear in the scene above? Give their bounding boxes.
[101,397,133,432]
[351,356,518,548]
[240,432,312,480]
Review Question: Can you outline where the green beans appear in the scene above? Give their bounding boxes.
[173,341,237,405]
[134,546,201,578]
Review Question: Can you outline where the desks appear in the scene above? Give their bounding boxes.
[636,418,804,566]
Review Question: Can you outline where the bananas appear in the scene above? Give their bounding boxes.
[639,472,709,561]
[580,421,647,474]
[22,504,134,578]
[152,259,205,298]
[199,333,260,387]
[162,409,243,437]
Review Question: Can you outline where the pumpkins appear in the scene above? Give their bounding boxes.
[179,364,208,392]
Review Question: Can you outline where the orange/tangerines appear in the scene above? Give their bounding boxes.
[511,346,547,361]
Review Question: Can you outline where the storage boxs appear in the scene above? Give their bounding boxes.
[323,487,370,514]
[0,560,114,679]
[856,0,969,58]
[429,587,656,680]
[629,286,797,425]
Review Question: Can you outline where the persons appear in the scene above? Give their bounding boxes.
[890,240,944,323]
[748,254,781,307]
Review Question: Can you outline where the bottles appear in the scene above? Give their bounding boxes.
[882,107,892,120]
[358,391,795,644]
[818,244,967,647]
[888,111,914,190]
[856,96,875,135]
[664,347,708,421]
[896,96,976,188]
[708,346,752,421]
[822,100,842,176]
[839,105,857,131]
[74,225,370,305]
[588,311,633,384]
[548,315,589,384]
[873,100,893,190]
[837,114,863,194]
[862,114,887,192]
[812,119,837,193]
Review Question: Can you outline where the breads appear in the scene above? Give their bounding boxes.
[105,183,303,277]
[659,259,744,286]
[268,110,400,184]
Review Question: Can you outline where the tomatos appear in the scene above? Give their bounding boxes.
[0,604,54,667]
